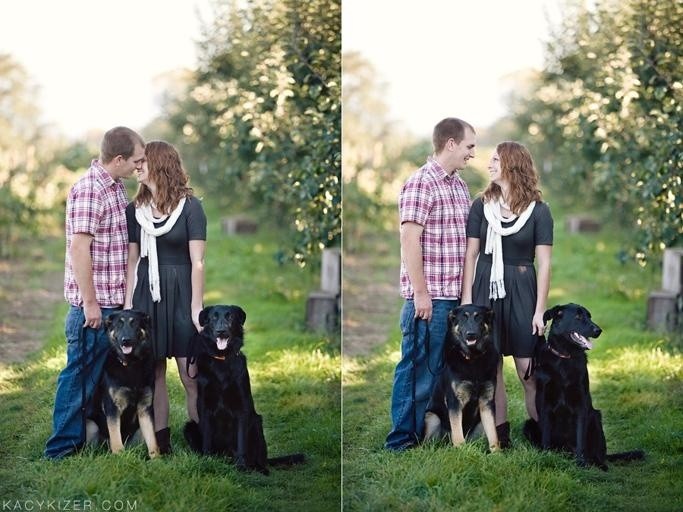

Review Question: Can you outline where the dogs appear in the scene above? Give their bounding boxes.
[423,304,501,453]
[524,302,646,471]
[86,310,160,461]
[184,305,310,476]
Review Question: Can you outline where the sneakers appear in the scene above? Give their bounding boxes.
[523,417,543,450]
[155,427,172,454]
[496,421,512,449]
[182,420,199,453]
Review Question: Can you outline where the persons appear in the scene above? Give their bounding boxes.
[460,139,558,453]
[384,118,477,452]
[43,125,145,462]
[123,139,208,462]
